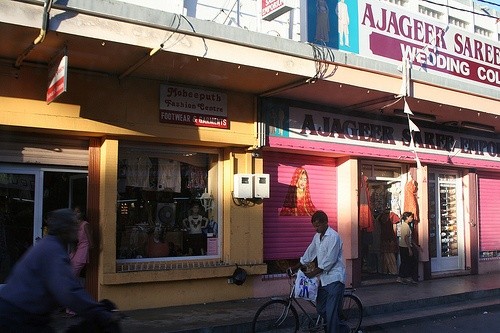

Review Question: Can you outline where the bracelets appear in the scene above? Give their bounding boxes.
[416,246,419,249]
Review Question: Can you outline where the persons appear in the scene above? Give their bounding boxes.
[287,211,345,333]
[395,210,424,285]
[0,209,132,333]
[66,209,94,316]
[182,203,207,255]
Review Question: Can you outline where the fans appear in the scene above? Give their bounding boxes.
[156,203,176,225]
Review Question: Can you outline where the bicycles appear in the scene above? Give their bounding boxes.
[0,299,128,333]
[251,267,364,333]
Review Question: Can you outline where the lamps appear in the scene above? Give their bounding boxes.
[231,263,248,285]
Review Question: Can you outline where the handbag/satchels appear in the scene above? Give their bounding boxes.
[294,268,319,306]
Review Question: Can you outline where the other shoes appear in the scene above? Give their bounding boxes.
[406,279,417,284]
[396,279,407,285]
